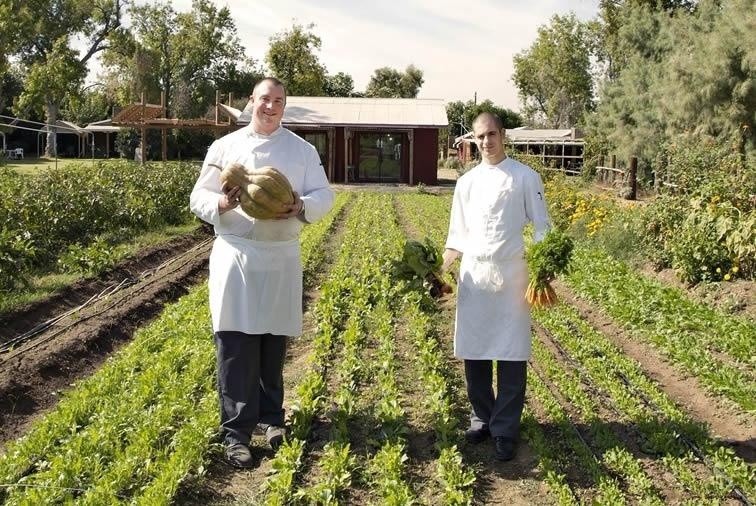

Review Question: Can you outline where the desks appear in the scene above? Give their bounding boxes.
[5,148,14,160]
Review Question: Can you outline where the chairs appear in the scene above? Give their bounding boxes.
[14,148,23,160]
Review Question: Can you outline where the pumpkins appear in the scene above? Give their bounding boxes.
[208,162,295,219]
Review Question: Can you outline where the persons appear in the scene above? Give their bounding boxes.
[425,110,555,461]
[188,76,337,469]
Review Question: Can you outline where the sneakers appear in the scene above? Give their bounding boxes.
[225,443,254,468]
[265,425,286,446]
[496,436,514,460]
[464,429,488,445]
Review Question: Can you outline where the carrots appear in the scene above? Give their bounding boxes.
[524,280,558,307]
